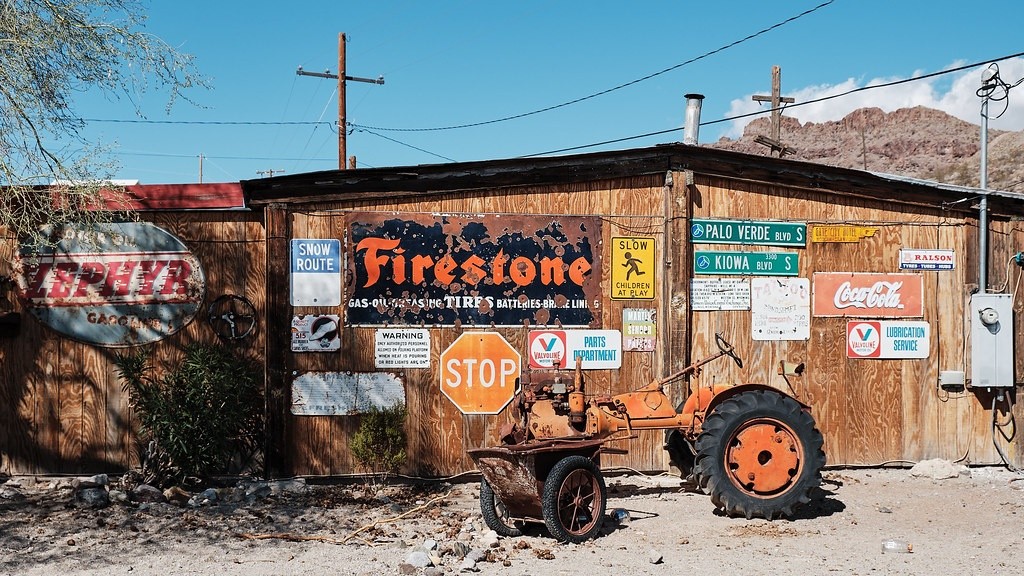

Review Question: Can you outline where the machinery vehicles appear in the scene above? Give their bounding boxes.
[501,329,827,523]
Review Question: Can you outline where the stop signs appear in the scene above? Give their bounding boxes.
[441,330,522,416]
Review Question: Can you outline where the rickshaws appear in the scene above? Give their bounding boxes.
[466,433,629,543]
